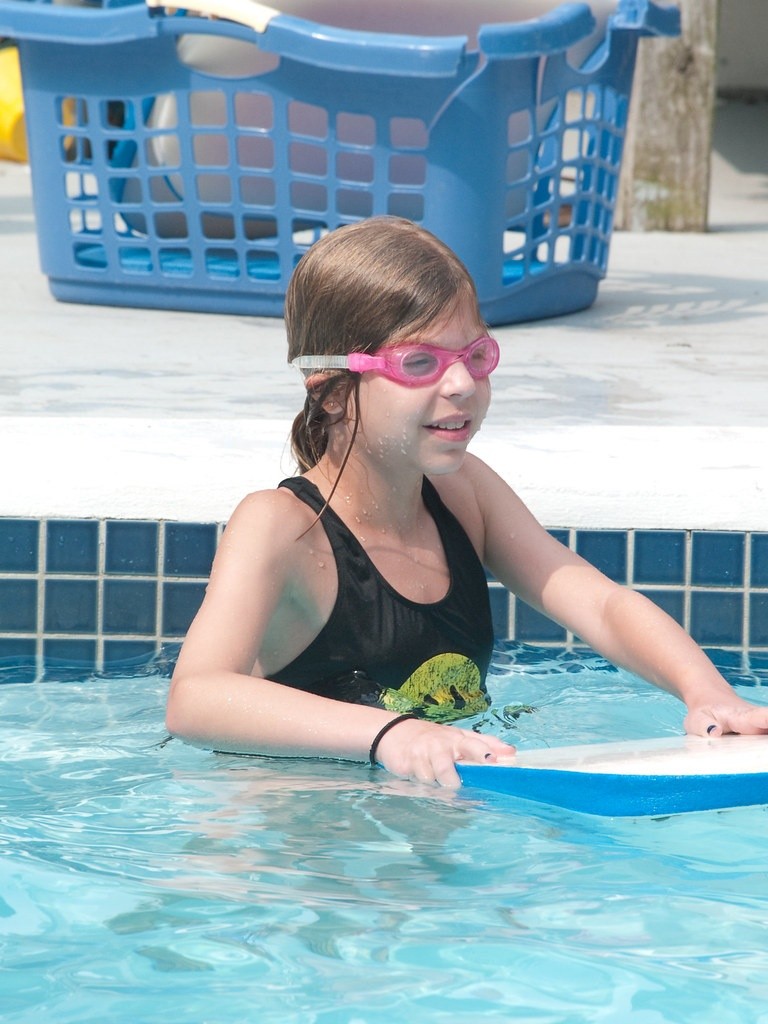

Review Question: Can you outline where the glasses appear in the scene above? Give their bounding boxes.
[350,336,501,389]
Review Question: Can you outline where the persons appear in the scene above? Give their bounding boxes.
[166,214,768,793]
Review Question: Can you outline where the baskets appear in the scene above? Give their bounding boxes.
[0,2,684,329]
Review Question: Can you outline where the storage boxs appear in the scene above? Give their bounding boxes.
[0,0,682,332]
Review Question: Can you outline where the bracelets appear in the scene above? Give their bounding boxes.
[368,714,419,766]
